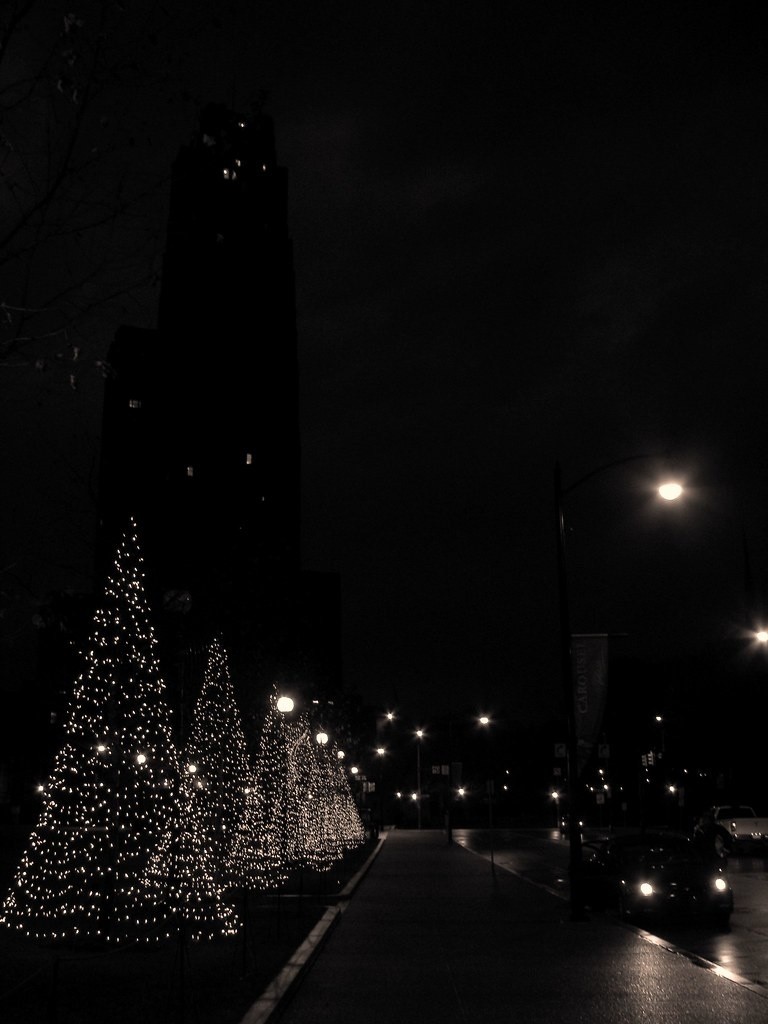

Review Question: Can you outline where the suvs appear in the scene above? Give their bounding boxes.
[698,803,768,861]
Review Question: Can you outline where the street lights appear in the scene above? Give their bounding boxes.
[415,730,424,830]
[377,711,394,832]
[479,716,494,829]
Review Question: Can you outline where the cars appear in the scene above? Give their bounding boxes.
[581,831,735,932]
